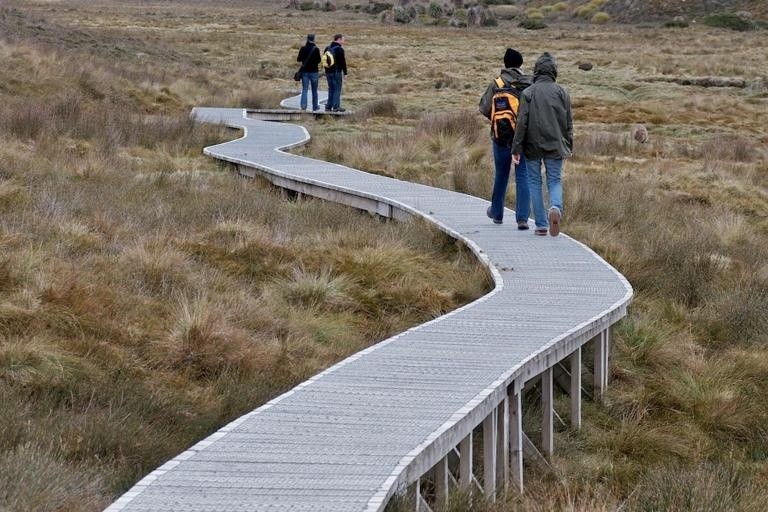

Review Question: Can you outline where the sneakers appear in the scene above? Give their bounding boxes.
[333,107,345,112]
[313,105,320,111]
[518,220,529,229]
[535,229,547,235]
[486,206,502,223]
[548,207,560,236]
[325,106,331,111]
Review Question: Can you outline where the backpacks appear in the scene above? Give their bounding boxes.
[321,46,338,70]
[490,77,519,146]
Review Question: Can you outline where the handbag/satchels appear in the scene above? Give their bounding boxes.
[293,72,301,81]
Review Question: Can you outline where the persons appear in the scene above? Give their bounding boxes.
[509,49,573,237]
[296,33,321,111]
[477,47,533,230]
[323,34,348,112]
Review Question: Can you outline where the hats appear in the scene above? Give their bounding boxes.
[503,47,523,68]
